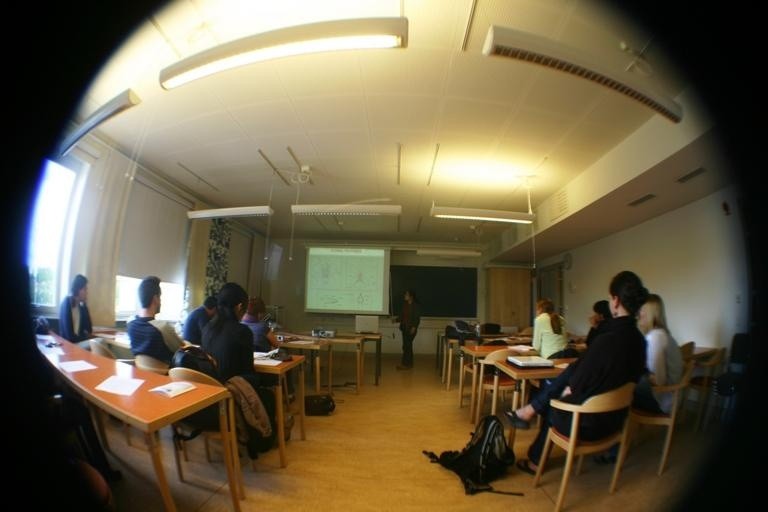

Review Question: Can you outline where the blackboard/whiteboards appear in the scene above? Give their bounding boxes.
[390,265,479,320]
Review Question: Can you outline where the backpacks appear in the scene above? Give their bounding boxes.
[301,393,335,418]
[462,412,517,486]
[169,344,216,380]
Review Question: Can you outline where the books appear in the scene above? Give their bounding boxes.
[148,380,197,399]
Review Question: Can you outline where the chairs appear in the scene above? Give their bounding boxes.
[88,339,290,501]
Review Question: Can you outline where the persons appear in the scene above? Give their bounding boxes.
[125,276,182,367]
[391,288,421,370]
[58,273,96,344]
[196,281,292,452]
[502,270,650,475]
[633,293,684,416]
[585,300,614,348]
[532,298,568,361]
[239,294,273,352]
[180,294,218,345]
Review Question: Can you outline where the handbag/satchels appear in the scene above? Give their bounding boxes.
[451,318,473,336]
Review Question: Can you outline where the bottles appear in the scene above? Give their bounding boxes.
[474,321,481,339]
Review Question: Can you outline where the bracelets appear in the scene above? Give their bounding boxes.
[412,326,416,330]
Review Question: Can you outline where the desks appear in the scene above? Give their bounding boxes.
[35,327,240,512]
[251,329,383,466]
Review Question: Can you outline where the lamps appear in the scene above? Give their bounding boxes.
[58,86,142,158]
[158,0,409,91]
[186,169,274,219]
[415,227,483,258]
[429,180,536,225]
[290,182,402,216]
[480,24,684,124]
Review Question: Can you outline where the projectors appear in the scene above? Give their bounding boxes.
[312,326,337,338]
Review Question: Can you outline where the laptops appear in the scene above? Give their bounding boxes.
[355,315,379,335]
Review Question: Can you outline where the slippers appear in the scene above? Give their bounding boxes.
[502,407,532,432]
[512,453,543,479]
